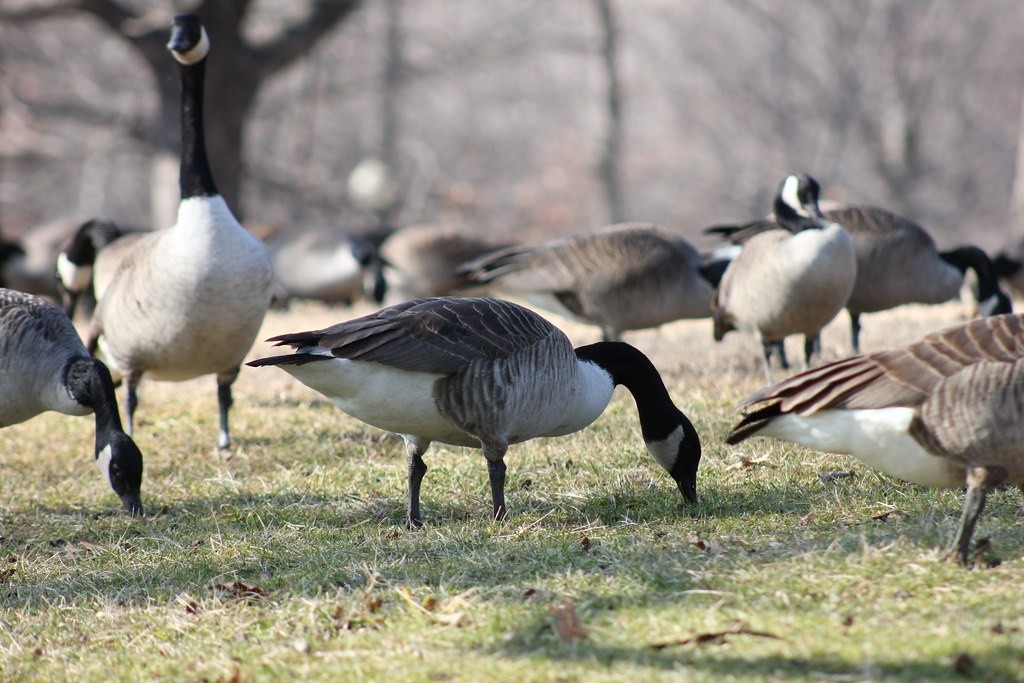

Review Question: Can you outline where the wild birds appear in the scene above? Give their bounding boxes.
[245,295,701,527]
[703,171,1024,377]
[723,313,1023,565]
[0,218,122,322]
[87,13,276,451]
[457,223,731,343]
[0,288,143,518]
[274,224,520,307]
